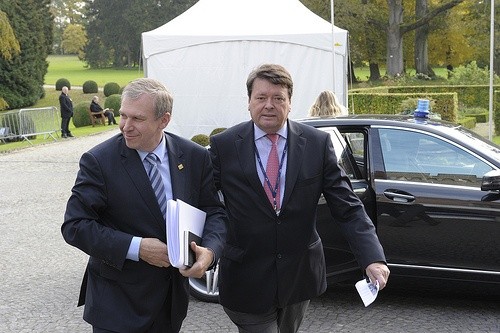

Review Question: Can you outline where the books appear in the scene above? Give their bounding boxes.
[183,231,202,268]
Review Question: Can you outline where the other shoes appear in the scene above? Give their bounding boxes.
[61,133,69,138]
[67,133,74,137]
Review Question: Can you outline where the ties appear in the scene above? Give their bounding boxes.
[142,153,167,221]
[263,134,281,209]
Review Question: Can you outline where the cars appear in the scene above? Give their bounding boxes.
[187,113,500,302]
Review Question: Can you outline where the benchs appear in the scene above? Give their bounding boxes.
[91,108,114,127]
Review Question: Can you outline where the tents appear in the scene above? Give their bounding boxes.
[140,0,349,150]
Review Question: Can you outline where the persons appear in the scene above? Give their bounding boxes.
[90,96,119,125]
[210,64,391,333]
[61,79,227,333]
[309,89,353,156]
[59,86,74,138]
